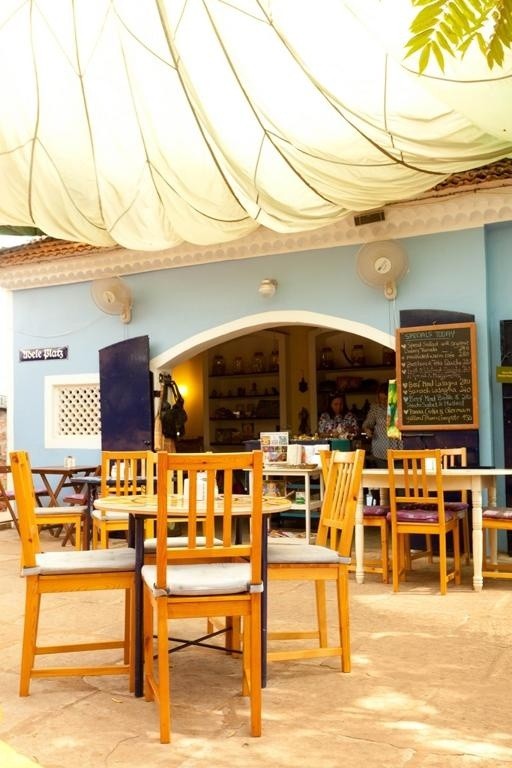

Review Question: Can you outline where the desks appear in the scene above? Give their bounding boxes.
[93,493,292,698]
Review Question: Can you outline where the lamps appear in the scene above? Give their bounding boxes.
[258,277,280,300]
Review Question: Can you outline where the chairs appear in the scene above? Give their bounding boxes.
[10,450,139,698]
[386,448,461,595]
[141,449,264,744]
[1,439,512,588]
[232,449,364,675]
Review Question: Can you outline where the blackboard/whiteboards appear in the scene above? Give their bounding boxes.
[393,320,482,431]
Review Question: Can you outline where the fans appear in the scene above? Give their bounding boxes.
[89,270,136,328]
[354,236,413,307]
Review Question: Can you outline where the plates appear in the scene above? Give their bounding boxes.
[297,464,317,469]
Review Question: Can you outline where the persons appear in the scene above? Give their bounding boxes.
[318,392,360,439]
[362,383,404,505]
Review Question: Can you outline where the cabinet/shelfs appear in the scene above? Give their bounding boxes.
[200,329,293,454]
[306,327,397,439]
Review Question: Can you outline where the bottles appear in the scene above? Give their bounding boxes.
[64,456,75,468]
[112,463,131,479]
[212,400,280,418]
[321,344,392,368]
[183,472,219,501]
[211,351,278,397]
